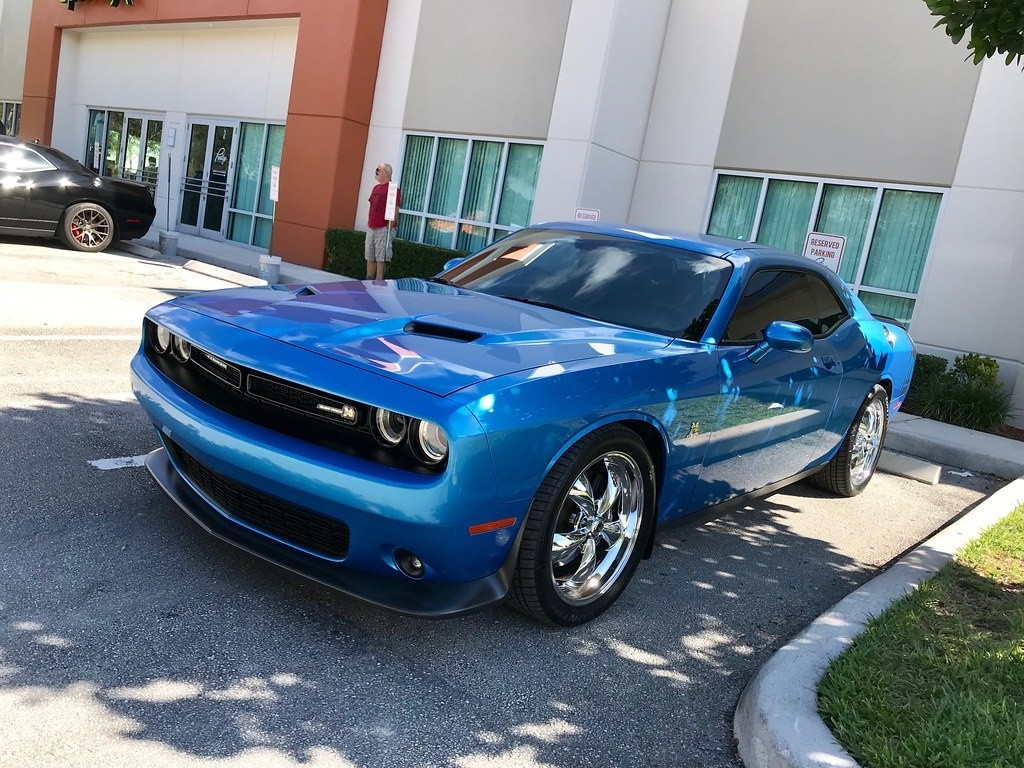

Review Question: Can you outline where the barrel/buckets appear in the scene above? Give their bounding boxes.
[258,254,283,285]
[157,229,180,256]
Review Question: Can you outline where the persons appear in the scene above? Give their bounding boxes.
[142,156,159,191]
[0,113,7,136]
[363,162,402,282]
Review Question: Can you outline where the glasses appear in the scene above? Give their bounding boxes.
[376,167,381,173]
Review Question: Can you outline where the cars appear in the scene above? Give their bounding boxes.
[0,134,158,254]
[126,216,920,631]
